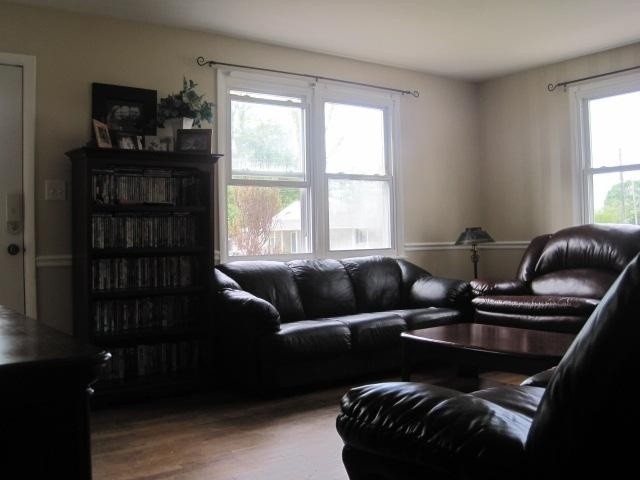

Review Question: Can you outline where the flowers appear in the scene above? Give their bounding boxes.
[153,76,216,128]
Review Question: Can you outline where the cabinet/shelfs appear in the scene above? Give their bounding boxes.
[64,147,224,411]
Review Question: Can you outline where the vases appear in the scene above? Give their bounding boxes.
[180,117,195,129]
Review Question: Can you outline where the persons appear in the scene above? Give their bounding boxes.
[105,105,142,131]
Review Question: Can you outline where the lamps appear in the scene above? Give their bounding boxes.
[454,227,495,279]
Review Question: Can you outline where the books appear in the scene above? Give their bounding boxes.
[90,165,209,384]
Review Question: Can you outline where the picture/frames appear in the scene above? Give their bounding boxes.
[91,82,176,155]
[176,129,212,154]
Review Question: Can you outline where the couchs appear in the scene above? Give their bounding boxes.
[210,256,473,403]
[337,252,640,480]
[469,223,640,334]
